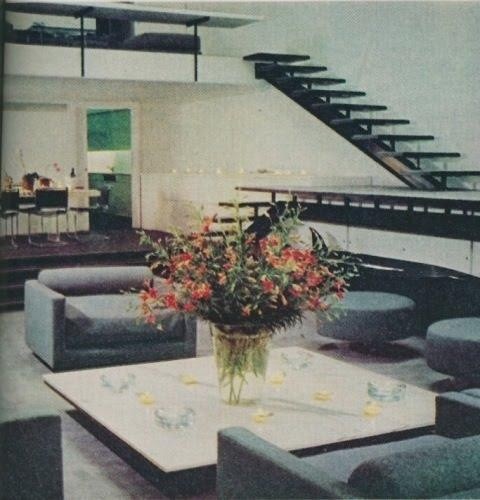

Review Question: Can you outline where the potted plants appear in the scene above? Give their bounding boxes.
[22,172,50,192]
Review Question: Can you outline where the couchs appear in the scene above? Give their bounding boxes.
[24,265,196,373]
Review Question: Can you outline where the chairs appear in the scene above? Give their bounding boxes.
[214,390,480,500]
[0,186,113,250]
[0,357,65,499]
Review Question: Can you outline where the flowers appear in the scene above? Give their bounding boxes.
[119,193,361,405]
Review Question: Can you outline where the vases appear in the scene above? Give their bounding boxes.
[209,322,278,407]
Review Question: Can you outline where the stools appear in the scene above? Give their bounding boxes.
[317,291,480,386]
